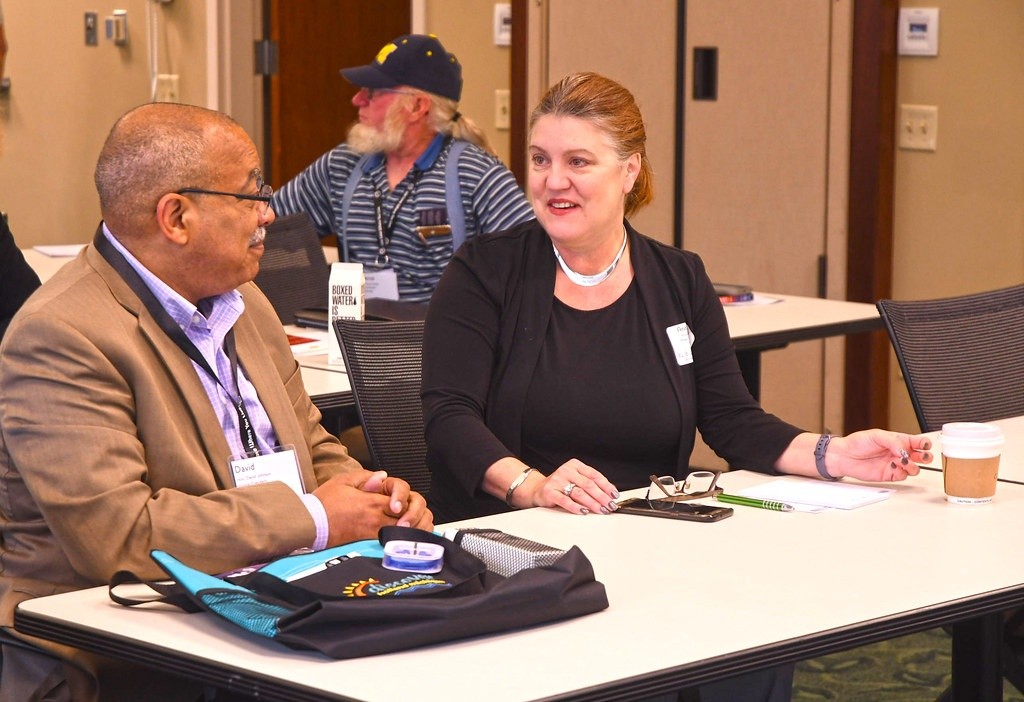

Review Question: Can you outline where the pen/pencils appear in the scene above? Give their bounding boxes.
[416,226,428,244]
[712,494,795,512]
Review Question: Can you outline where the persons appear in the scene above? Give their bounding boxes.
[418,70,933,527]
[263,34,537,307]
[1,101,433,702]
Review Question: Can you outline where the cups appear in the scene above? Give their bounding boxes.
[938,421,1005,504]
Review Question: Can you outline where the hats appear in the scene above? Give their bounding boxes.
[340,32,462,103]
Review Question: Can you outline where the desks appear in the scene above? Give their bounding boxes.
[20,241,877,457]
[12,458,1024,702]
[892,415,1024,488]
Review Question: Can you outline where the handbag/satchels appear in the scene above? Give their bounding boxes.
[110,516,608,659]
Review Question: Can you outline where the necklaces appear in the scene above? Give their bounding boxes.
[552,224,627,287]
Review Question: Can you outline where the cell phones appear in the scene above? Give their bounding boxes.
[611,497,733,523]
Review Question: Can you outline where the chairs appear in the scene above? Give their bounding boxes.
[332,318,437,499]
[875,281,1024,702]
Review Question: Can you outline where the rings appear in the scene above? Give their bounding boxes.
[563,483,577,495]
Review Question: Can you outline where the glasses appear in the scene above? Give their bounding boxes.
[646,464,724,510]
[176,173,274,215]
[357,83,418,102]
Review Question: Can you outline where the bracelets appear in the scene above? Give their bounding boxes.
[813,434,847,482]
[505,467,538,509]
